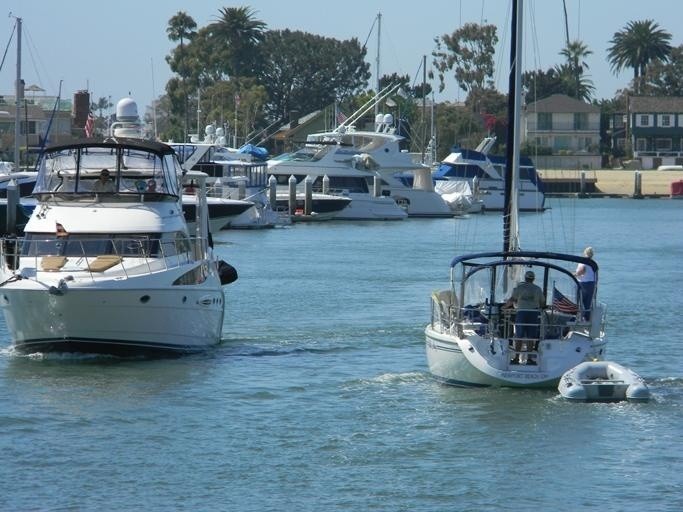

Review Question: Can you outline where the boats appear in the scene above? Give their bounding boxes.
[556,358,653,405]
[0,97,238,359]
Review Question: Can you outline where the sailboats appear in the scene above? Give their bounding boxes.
[423,0,609,390]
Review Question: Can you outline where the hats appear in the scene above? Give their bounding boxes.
[525,272,534,279]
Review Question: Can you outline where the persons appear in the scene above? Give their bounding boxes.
[145,180,159,193]
[501,271,545,365]
[573,247,598,321]
[91,169,115,193]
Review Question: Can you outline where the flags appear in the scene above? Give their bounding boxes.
[552,287,578,314]
[337,106,347,124]
[85,111,95,138]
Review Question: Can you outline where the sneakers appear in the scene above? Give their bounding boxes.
[527,359,536,364]
[511,358,520,365]
